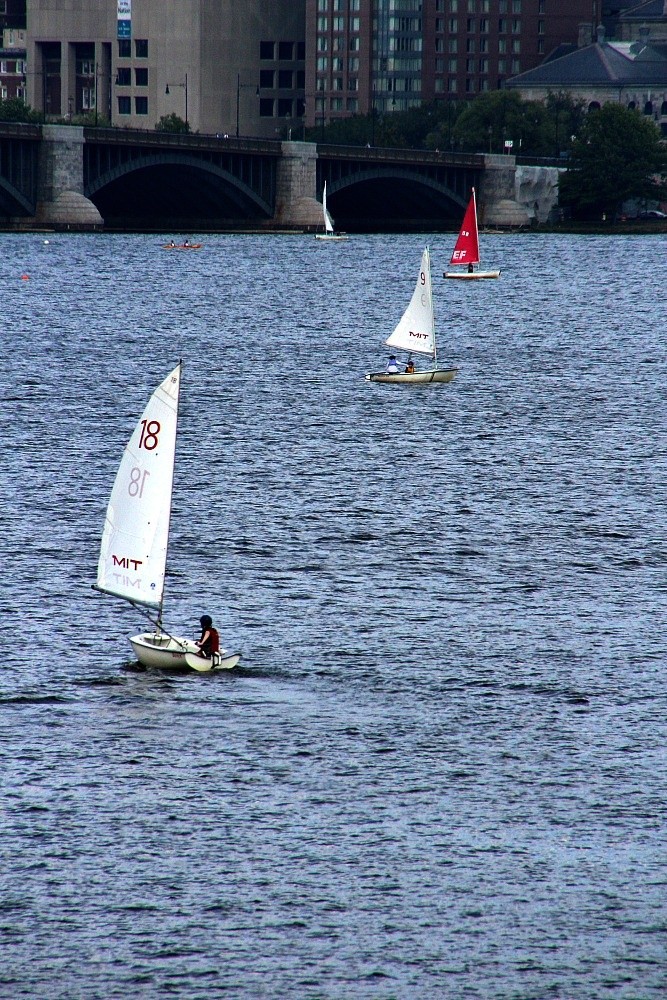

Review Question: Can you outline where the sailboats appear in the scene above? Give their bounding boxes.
[314,179,350,243]
[439,185,501,281]
[365,246,457,386]
[92,364,243,673]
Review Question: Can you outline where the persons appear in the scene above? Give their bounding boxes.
[184,240,189,247]
[405,361,415,375]
[170,240,174,247]
[463,262,473,273]
[385,355,409,375]
[193,615,220,659]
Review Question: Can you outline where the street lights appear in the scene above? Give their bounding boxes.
[165,73,189,128]
[235,72,260,137]
[371,82,397,147]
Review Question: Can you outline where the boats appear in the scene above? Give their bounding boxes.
[162,241,201,250]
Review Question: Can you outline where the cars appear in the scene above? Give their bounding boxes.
[599,209,667,231]
